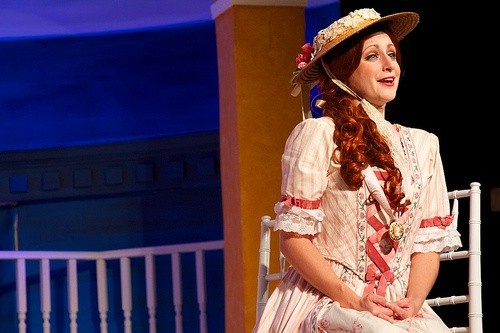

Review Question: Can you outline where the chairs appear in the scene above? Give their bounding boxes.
[255,215,292,324]
[423,180,484,333]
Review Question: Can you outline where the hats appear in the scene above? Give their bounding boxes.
[290,8,419,85]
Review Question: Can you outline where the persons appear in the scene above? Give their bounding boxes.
[253,8,462,333]
